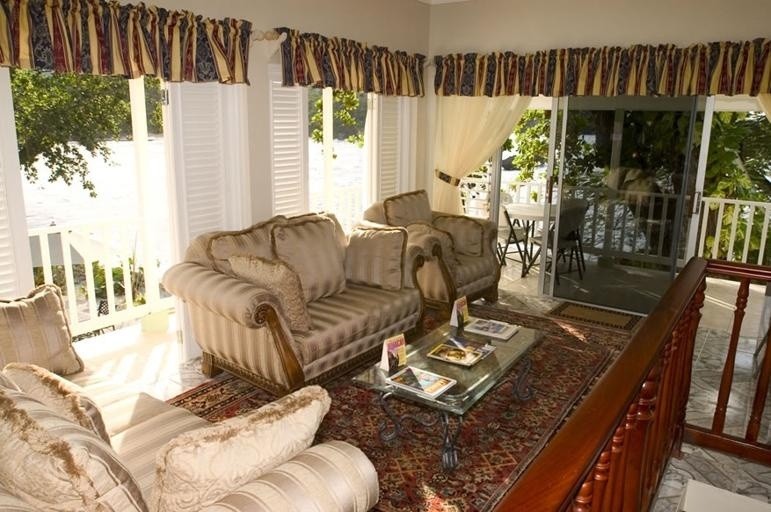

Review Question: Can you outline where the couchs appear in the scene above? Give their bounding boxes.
[0,367,381,512]
[161,230,424,398]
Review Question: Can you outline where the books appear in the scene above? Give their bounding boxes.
[384,316,522,399]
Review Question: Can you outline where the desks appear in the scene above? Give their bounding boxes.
[676,479,771,512]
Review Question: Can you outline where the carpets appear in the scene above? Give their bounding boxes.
[165,301,632,512]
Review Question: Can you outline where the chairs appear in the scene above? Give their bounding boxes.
[363,202,500,311]
[465,190,588,285]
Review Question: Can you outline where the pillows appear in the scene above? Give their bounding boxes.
[0,283,84,377]
[152,384,331,511]
[383,190,485,278]
[207,209,408,331]
